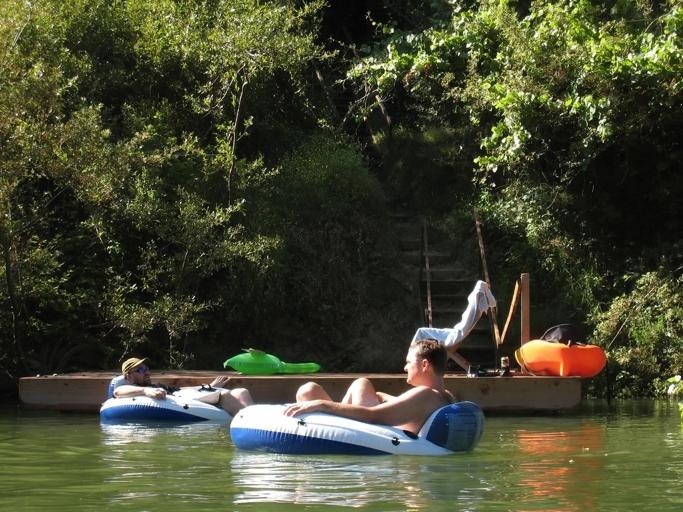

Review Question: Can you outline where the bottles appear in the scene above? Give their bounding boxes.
[500,353,510,377]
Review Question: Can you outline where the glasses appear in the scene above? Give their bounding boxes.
[128,365,151,374]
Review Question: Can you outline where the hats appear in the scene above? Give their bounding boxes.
[122,356,154,379]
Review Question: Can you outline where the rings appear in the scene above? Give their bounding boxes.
[218,377,221,380]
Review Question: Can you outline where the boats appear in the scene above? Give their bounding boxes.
[510,318,610,381]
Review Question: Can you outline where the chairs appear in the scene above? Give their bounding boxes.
[407,279,489,378]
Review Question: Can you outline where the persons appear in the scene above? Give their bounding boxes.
[249,457,439,512]
[112,355,255,417]
[282,339,456,435]
